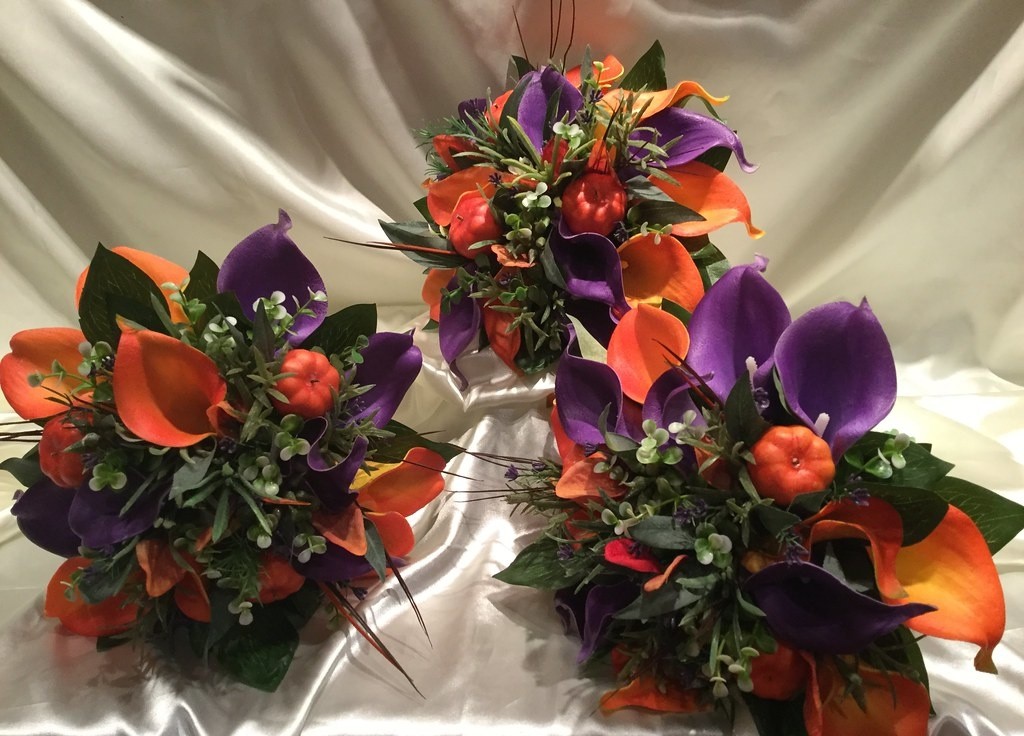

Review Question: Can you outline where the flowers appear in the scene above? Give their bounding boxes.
[1,205,479,699]
[431,253,1024,736]
[323,0,767,391]
